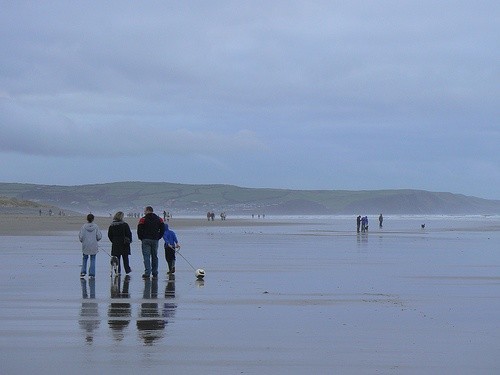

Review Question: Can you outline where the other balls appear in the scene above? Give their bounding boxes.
[195,269,206,279]
[195,279,205,288]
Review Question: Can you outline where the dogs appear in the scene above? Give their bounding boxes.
[421,224,425,228]
[110,256,119,277]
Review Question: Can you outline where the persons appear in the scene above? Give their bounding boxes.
[162,224,180,274]
[129,211,170,222]
[356,215,368,233]
[108,211,132,275]
[79,214,102,279]
[137,206,164,278]
[379,213,384,227]
[80,273,177,347]
[207,211,226,222]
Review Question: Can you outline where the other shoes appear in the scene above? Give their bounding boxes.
[80,274,85,276]
[167,271,174,274]
[143,274,150,277]
